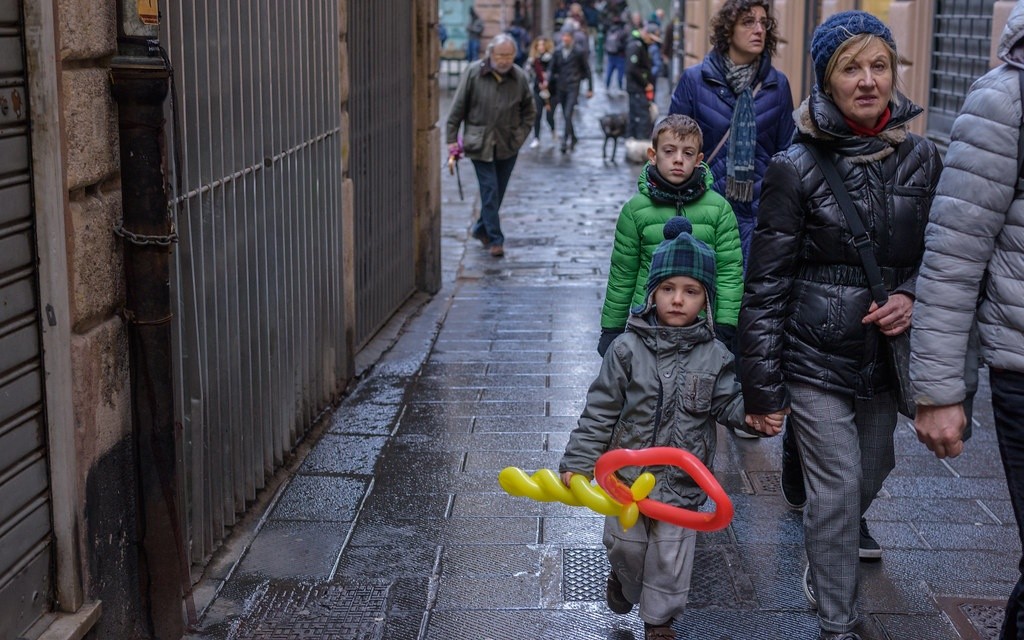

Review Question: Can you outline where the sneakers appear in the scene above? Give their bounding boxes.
[645,619,676,640]
[781,440,809,508]
[607,565,634,613]
[857,519,883,558]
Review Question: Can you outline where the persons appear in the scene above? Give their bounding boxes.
[439,10,447,47]
[669,1,794,437]
[506,0,684,153]
[467,8,483,62]
[598,116,743,358]
[780,414,882,558]
[736,11,941,640]
[909,0,1024,639]
[560,216,791,640]
[446,35,535,255]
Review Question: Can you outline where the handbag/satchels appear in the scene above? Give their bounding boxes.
[892,331,916,420]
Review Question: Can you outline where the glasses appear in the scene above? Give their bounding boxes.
[738,17,774,30]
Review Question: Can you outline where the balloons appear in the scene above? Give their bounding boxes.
[498,447,734,531]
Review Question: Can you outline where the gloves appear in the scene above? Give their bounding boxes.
[596,333,620,357]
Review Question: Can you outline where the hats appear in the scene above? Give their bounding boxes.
[810,11,897,89]
[630,215,716,335]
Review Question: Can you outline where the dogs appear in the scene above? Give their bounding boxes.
[599,113,630,161]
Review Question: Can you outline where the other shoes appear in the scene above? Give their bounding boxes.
[819,625,859,640]
[478,234,490,249]
[489,245,503,256]
[529,140,540,148]
[803,559,819,604]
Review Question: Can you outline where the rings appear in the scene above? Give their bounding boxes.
[754,421,760,424]
[890,324,894,329]
